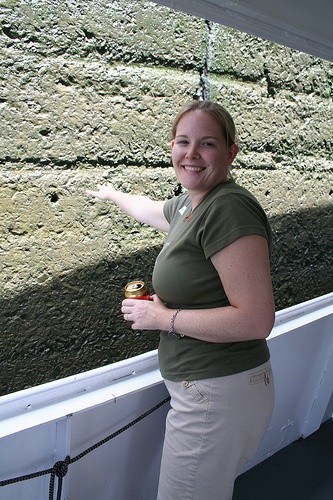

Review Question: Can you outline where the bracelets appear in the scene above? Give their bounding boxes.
[168,308,185,338]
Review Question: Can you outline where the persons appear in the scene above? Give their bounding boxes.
[84,100,278,500]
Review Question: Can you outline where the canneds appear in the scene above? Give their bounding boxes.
[124,280,150,300]
